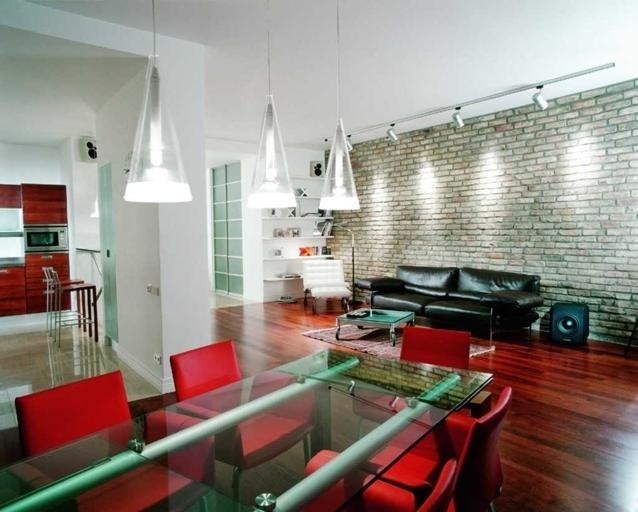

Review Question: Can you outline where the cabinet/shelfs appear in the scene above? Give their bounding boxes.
[0,184,21,209]
[263,174,335,283]
[22,254,71,314]
[0,267,27,317]
[20,183,67,224]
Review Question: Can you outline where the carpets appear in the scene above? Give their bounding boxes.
[301,322,495,363]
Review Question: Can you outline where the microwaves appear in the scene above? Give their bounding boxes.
[24,227,69,251]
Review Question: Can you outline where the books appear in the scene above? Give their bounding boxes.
[298,246,319,256]
[311,220,332,236]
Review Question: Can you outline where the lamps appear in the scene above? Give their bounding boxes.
[318,1,360,212]
[452,107,466,130]
[122,1,192,204]
[386,123,399,142]
[247,31,296,210]
[531,84,549,111]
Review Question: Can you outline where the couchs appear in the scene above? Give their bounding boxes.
[356,263,544,348]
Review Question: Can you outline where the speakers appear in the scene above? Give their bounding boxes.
[549,304,590,346]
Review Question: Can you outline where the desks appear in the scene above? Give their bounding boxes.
[1,353,496,511]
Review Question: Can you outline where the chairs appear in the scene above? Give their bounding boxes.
[10,369,219,510]
[301,259,353,315]
[158,338,317,512]
[302,454,458,510]
[41,265,98,349]
[353,326,470,440]
[358,384,513,512]
